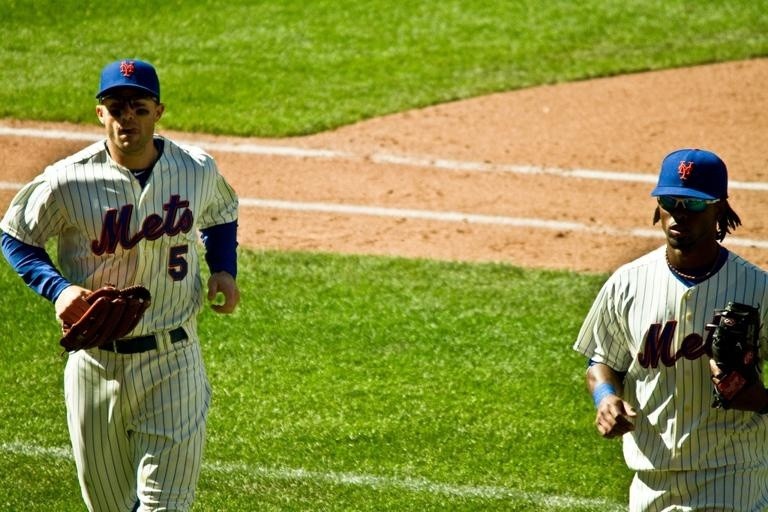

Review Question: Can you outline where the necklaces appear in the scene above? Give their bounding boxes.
[665,247,718,281]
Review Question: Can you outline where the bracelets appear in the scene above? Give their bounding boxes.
[592,383,616,409]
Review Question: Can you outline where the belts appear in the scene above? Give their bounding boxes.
[98,327,187,353]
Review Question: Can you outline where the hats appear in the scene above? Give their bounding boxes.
[651,149,727,199]
[96,60,159,98]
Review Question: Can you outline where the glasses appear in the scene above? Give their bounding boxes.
[656,196,720,212]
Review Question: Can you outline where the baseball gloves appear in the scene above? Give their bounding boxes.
[705,301,768,412]
[59,282,152,357]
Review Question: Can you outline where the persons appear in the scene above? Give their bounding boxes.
[0,58,242,512]
[572,148,768,512]
